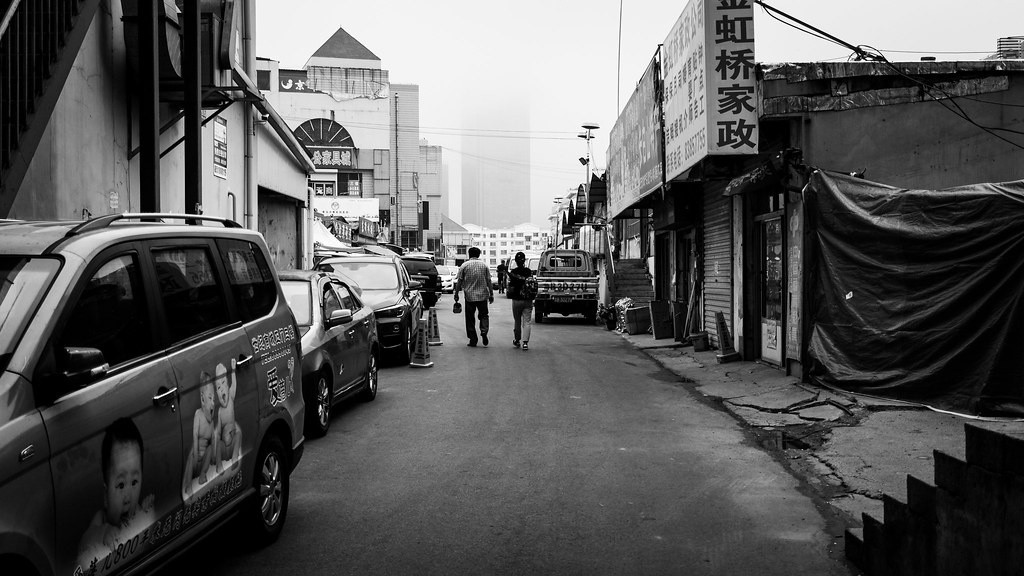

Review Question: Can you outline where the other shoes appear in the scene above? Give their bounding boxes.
[523,344,528,349]
[513,340,520,347]
[482,332,488,345]
[467,342,476,346]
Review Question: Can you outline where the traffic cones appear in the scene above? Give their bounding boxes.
[410,319,435,368]
[426,305,444,347]
[713,309,740,364]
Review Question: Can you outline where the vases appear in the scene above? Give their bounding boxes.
[689,331,710,350]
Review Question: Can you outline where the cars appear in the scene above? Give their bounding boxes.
[276,267,381,439]
[434,264,460,294]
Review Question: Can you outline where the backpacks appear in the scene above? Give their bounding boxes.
[513,270,539,300]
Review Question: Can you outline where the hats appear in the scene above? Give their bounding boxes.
[515,252,526,260]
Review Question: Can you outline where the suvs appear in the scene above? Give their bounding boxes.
[307,250,425,367]
[0,209,307,576]
[399,255,443,311]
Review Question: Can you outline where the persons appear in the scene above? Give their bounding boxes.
[453,247,494,346]
[495,260,509,294]
[507,252,534,350]
[71,357,244,576]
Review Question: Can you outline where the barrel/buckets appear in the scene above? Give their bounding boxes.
[689,331,710,352]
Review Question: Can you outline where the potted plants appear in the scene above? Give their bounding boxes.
[594,300,617,333]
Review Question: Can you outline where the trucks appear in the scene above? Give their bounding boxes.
[488,254,541,298]
[532,248,600,324]
